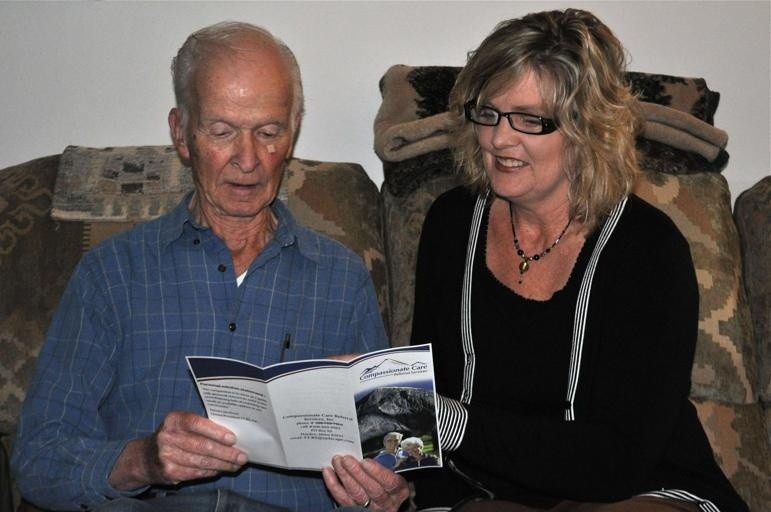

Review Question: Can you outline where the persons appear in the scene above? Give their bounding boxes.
[371,432,410,472]
[325,7,750,512]
[7,20,410,512]
[394,437,439,472]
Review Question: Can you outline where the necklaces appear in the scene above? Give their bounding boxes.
[508,202,578,285]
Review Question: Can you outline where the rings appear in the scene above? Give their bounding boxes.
[362,499,371,509]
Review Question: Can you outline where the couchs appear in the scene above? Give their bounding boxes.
[1,150,771,512]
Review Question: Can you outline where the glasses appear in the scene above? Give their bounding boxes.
[463,97,577,135]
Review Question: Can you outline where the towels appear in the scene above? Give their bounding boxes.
[48,145,290,224]
